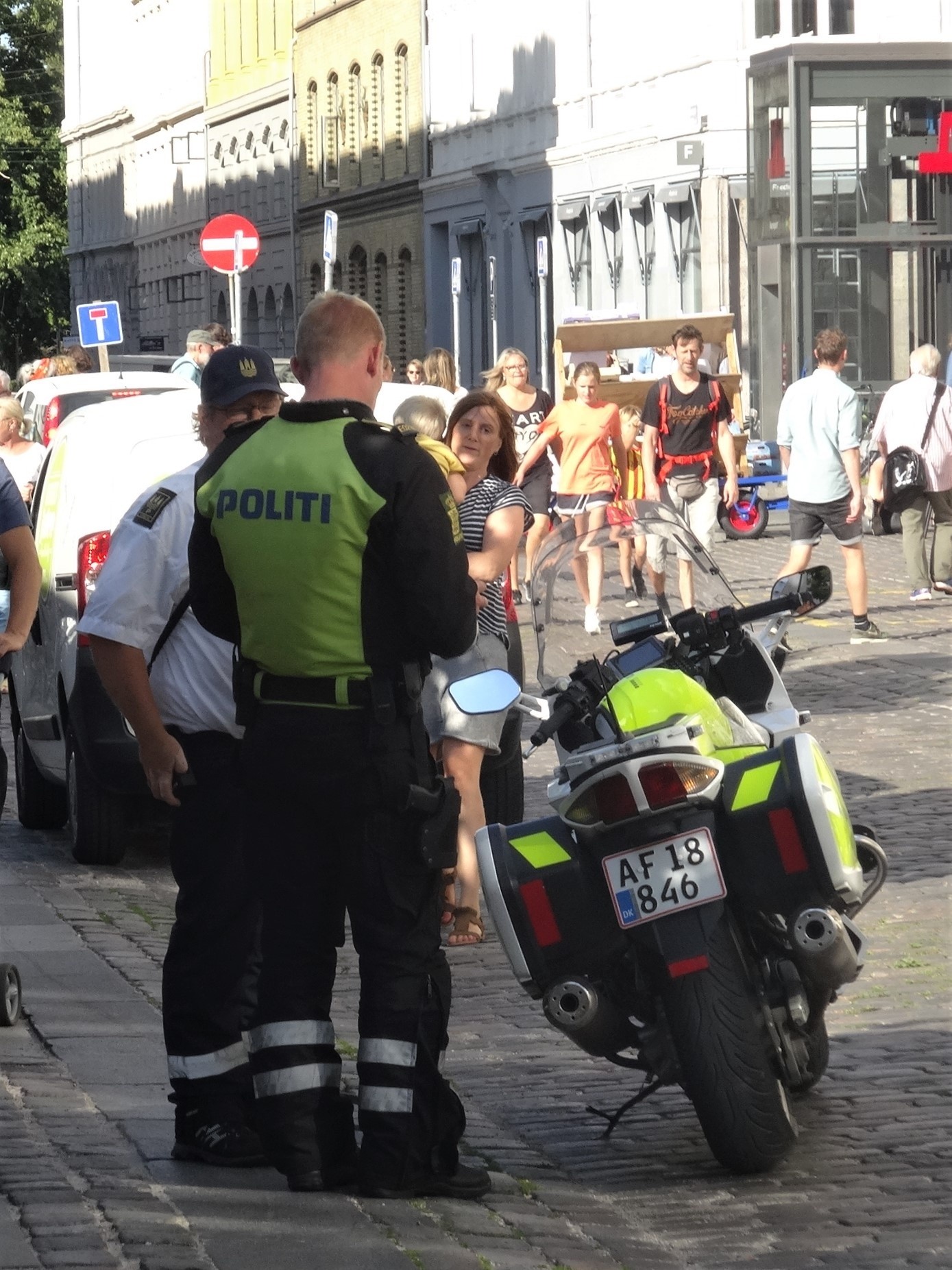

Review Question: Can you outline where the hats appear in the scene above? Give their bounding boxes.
[187,330,220,346]
[200,345,289,406]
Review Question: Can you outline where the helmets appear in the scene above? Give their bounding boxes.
[592,667,734,749]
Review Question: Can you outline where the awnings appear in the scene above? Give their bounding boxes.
[450,180,700,297]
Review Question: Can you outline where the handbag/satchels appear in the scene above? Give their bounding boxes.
[882,446,927,512]
[670,473,704,497]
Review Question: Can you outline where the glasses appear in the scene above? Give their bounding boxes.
[410,371,420,374]
[218,401,278,419]
[505,364,527,372]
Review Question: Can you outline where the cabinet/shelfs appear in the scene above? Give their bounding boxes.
[553,314,753,478]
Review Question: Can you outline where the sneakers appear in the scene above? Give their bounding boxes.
[361,1151,491,1198]
[850,622,889,645]
[768,622,793,653]
[933,578,952,594]
[288,1147,360,1190]
[168,1092,279,1164]
[910,587,932,601]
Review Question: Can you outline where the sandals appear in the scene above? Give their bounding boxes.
[447,906,484,946]
[439,866,458,926]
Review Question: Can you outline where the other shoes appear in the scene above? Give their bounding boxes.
[632,574,647,599]
[656,594,676,635]
[585,605,600,635]
[522,578,541,606]
[511,589,522,605]
[625,590,639,608]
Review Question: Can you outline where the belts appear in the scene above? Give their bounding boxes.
[260,676,370,705]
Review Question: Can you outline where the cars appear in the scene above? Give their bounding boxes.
[9,371,217,515]
[5,380,471,866]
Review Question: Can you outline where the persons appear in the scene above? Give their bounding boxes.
[172,330,220,387]
[187,287,494,1199]
[406,358,422,385]
[381,354,396,382]
[0,459,43,657]
[609,405,648,606]
[513,361,628,634]
[201,322,233,350]
[0,396,47,513]
[0,345,93,398]
[78,343,289,1166]
[769,332,889,653]
[392,396,468,503]
[641,326,740,616]
[867,343,952,599]
[481,347,564,607]
[420,347,469,399]
[434,390,534,946]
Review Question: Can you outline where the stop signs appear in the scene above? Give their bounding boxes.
[200,213,261,276]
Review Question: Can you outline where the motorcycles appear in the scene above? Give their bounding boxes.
[447,500,890,1174]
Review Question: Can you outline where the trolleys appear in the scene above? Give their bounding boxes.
[720,384,884,540]
